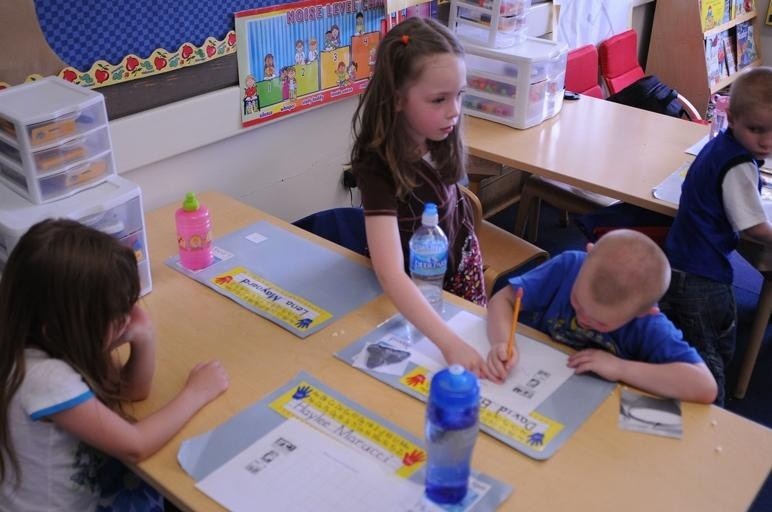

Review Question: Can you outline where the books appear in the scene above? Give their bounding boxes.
[705,21,759,85]
[700,0,756,32]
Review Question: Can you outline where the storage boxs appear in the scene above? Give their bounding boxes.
[462,38,568,129]
[448,0,529,48]
[1,77,117,204]
[1,175,152,302]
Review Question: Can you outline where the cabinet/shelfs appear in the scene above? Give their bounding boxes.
[645,0,760,123]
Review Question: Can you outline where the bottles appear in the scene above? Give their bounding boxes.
[409,204,449,341]
[710,96,730,142]
[425,365,481,505]
[175,192,214,270]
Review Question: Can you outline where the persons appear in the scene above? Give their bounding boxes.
[349,16,502,384]
[485,228,718,404]
[666,65,771,365]
[1,216,229,511]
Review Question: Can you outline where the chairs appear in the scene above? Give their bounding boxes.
[461,184,549,303]
[514,87,700,240]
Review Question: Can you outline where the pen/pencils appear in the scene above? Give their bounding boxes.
[506,286,523,358]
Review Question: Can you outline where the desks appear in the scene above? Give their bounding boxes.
[458,93,772,401]
[0,190,772,512]
